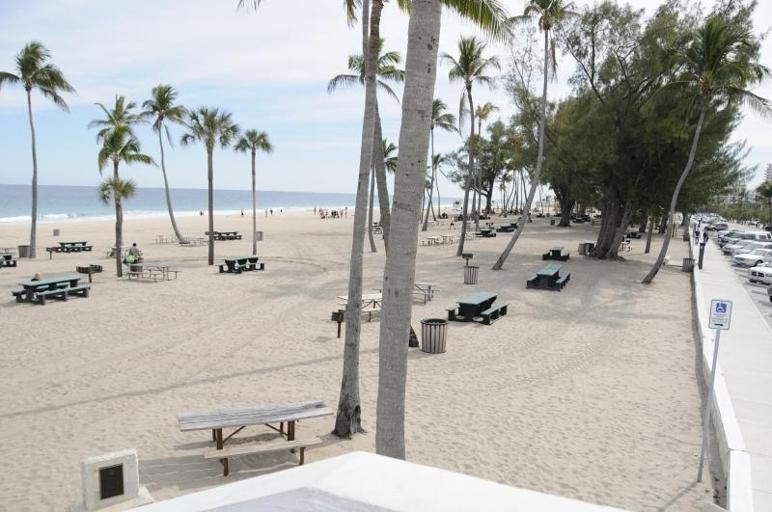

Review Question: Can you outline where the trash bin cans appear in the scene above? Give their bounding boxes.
[683,235,688,241]
[256,231,263,241]
[551,220,555,225]
[18,245,30,257]
[579,244,585,255]
[682,258,695,272]
[420,318,448,353]
[464,266,479,285]
[53,229,59,236]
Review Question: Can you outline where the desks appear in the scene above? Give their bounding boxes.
[215,232,237,240]
[414,280,436,288]
[59,241,88,252]
[456,292,496,324]
[129,262,172,282]
[550,247,563,259]
[178,400,334,474]
[18,275,81,304]
[223,256,259,273]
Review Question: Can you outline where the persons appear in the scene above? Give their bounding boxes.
[693,218,708,244]
[129,243,140,277]
[265,208,283,219]
[442,206,524,231]
[314,207,349,220]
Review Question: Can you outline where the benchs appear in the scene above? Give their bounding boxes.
[206,435,323,476]
[53,246,93,252]
[337,287,440,316]
[216,264,265,273]
[12,284,91,305]
[527,251,570,291]
[128,270,180,282]
[446,305,507,325]
[214,235,242,240]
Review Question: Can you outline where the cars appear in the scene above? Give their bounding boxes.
[693,213,728,231]
[718,226,772,285]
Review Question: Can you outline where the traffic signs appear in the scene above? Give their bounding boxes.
[709,300,733,330]
